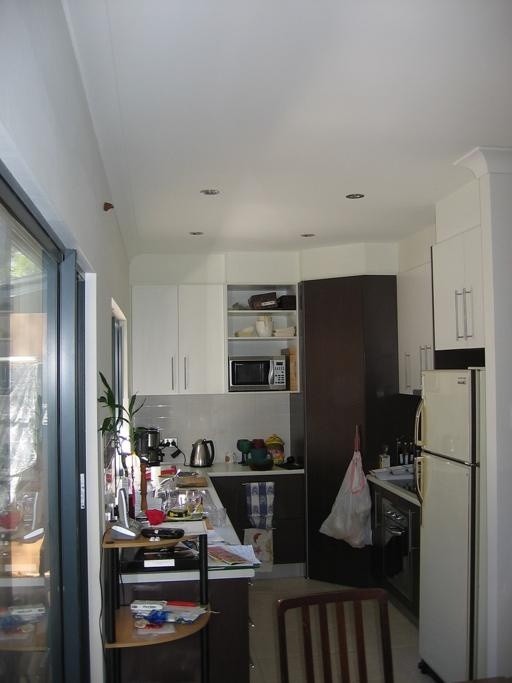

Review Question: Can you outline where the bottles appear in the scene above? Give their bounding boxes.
[379,441,415,469]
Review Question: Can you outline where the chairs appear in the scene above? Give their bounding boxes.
[274,589,394,683]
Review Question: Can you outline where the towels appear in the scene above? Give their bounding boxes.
[245,481,276,531]
[242,529,274,576]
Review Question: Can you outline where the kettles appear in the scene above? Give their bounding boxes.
[189,438,214,467]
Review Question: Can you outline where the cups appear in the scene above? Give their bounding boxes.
[254,315,273,338]
[161,487,227,528]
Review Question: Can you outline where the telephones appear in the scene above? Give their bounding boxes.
[112,488,143,540]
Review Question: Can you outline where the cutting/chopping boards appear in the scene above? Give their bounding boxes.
[176,476,208,487]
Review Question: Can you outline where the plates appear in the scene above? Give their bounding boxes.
[271,326,296,337]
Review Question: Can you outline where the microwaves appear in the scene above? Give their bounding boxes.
[228,355,290,393]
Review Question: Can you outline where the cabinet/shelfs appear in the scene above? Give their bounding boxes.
[102,526,211,683]
[213,474,305,565]
[227,281,301,395]
[431,224,486,350]
[131,284,227,395]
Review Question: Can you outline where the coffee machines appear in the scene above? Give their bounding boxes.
[147,427,163,466]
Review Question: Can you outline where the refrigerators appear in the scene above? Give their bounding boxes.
[415,367,487,683]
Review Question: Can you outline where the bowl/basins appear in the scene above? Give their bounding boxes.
[248,459,274,471]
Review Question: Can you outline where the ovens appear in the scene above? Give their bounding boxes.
[372,483,419,626]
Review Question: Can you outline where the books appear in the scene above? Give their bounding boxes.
[143,552,173,567]
[207,544,261,571]
